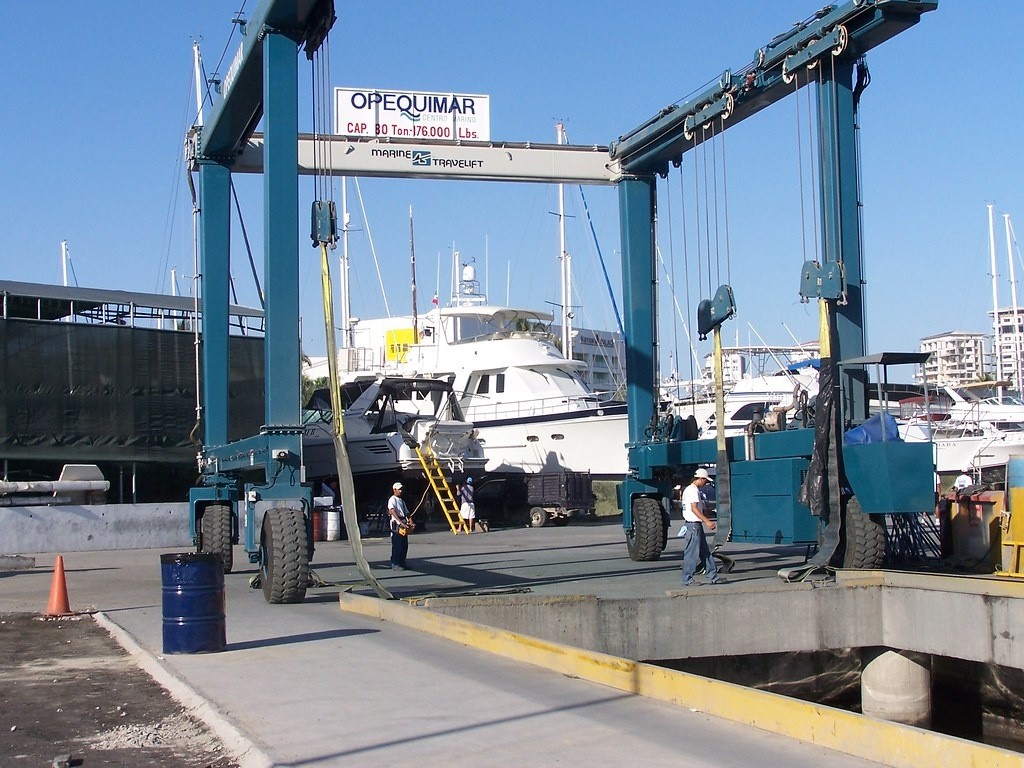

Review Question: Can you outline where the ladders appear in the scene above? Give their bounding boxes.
[410,446,469,535]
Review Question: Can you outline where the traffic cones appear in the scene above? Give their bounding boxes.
[45,556,75,616]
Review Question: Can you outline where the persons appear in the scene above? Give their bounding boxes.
[682,468,727,586]
[456,477,476,533]
[387,482,416,571]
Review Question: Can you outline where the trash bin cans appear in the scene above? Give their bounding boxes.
[160,552,226,655]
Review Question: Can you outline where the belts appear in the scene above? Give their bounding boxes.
[686,520,701,523]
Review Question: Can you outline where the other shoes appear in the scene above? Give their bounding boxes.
[935,522,940,526]
[687,581,701,586]
[405,566,412,570]
[714,578,728,583]
[392,565,404,571]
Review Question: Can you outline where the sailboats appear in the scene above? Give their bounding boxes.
[301,117,1024,518]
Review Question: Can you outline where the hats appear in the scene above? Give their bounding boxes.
[393,482,405,490]
[961,466,967,472]
[694,469,713,481]
[467,477,473,483]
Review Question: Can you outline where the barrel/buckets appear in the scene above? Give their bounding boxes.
[321,511,340,541]
[160,550,226,655]
